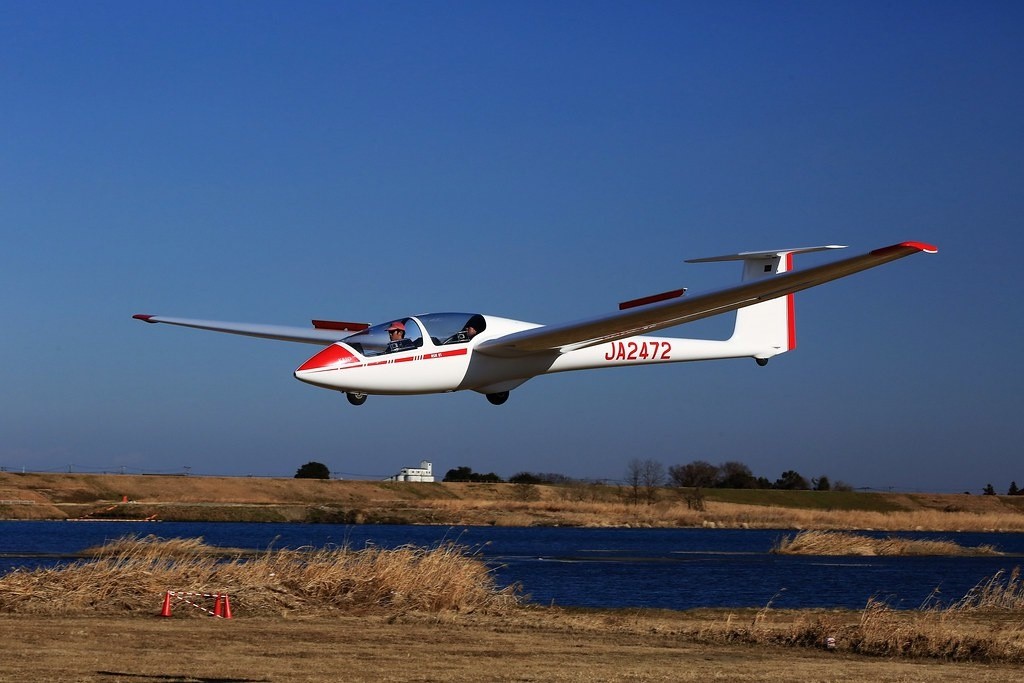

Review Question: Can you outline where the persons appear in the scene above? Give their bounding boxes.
[366,322,406,357]
[465,321,482,340]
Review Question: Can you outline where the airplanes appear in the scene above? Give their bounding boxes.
[131,240,938,406]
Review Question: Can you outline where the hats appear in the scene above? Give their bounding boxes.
[385,322,405,332]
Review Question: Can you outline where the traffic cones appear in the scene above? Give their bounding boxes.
[224,593,232,619]
[159,590,172,616]
[212,594,222,616]
